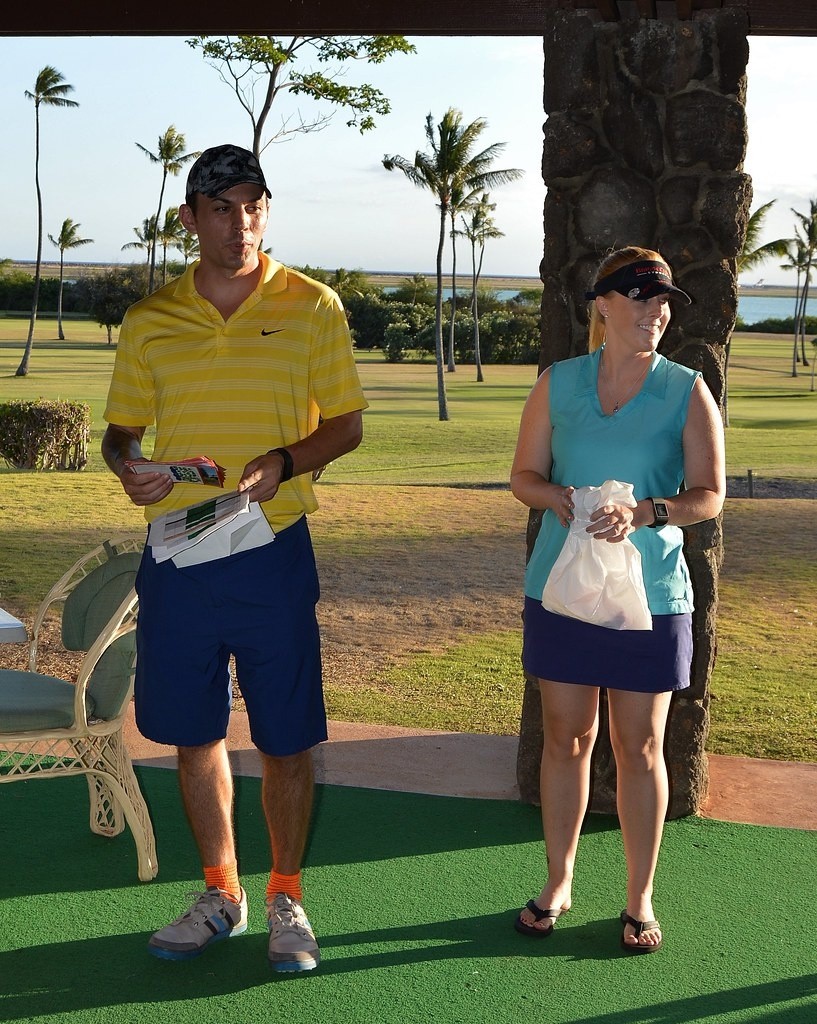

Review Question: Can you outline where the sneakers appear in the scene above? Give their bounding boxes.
[148,885,248,961]
[265,892,321,972]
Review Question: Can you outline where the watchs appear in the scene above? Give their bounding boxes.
[645,497,670,528]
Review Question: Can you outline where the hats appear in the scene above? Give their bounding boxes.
[584,260,692,307]
[185,144,272,201]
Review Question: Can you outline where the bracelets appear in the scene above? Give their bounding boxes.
[266,448,294,482]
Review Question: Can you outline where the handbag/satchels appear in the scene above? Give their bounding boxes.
[540,477,653,630]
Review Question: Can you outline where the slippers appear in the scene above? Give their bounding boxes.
[620,908,661,952]
[514,898,566,939]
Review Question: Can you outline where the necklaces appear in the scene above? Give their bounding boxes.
[600,349,653,412]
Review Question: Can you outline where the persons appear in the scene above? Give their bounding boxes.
[102,141,370,972]
[511,246,727,951]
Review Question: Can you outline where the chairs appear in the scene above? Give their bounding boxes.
[0,534,160,882]
